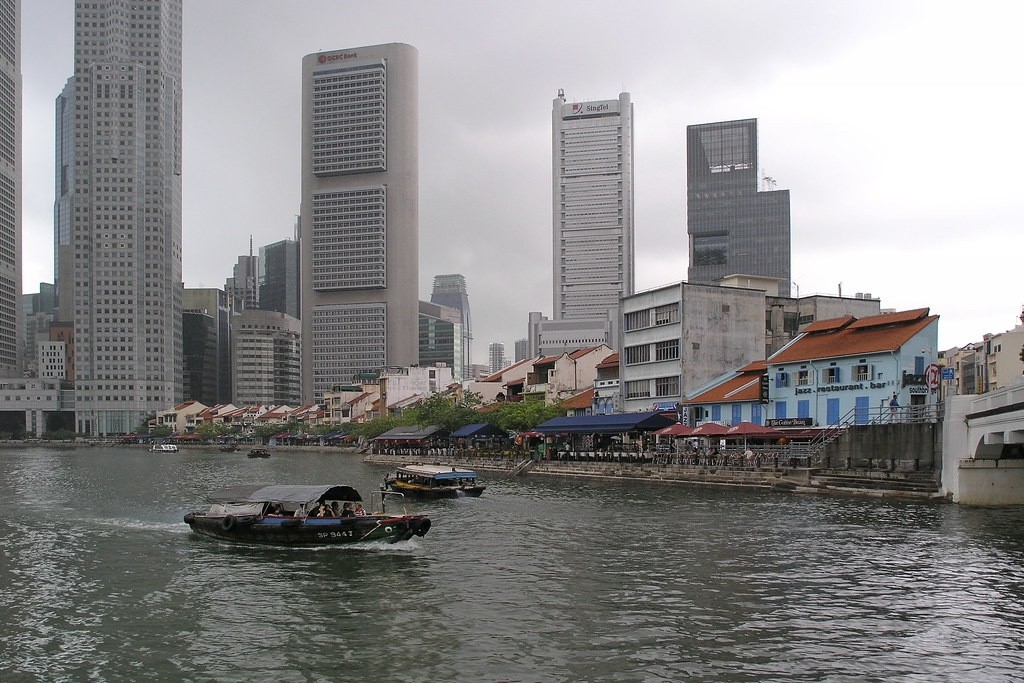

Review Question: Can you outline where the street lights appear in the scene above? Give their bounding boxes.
[921,345,932,422]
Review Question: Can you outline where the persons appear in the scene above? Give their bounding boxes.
[294,504,309,517]
[741,448,754,462]
[687,439,698,453]
[565,443,571,460]
[317,502,366,517]
[408,475,428,485]
[698,447,720,459]
[267,504,283,517]
[451,478,475,486]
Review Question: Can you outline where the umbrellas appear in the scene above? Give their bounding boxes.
[652,421,784,457]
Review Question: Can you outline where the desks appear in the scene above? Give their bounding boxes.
[557,451,652,463]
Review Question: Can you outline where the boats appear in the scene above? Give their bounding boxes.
[247,449,271,458]
[384,465,486,503]
[148,444,179,452]
[219,444,241,452]
[182,485,432,548]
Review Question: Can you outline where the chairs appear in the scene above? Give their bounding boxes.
[651,452,756,466]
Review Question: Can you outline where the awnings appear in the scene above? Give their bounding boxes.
[271,432,346,445]
[532,412,677,436]
